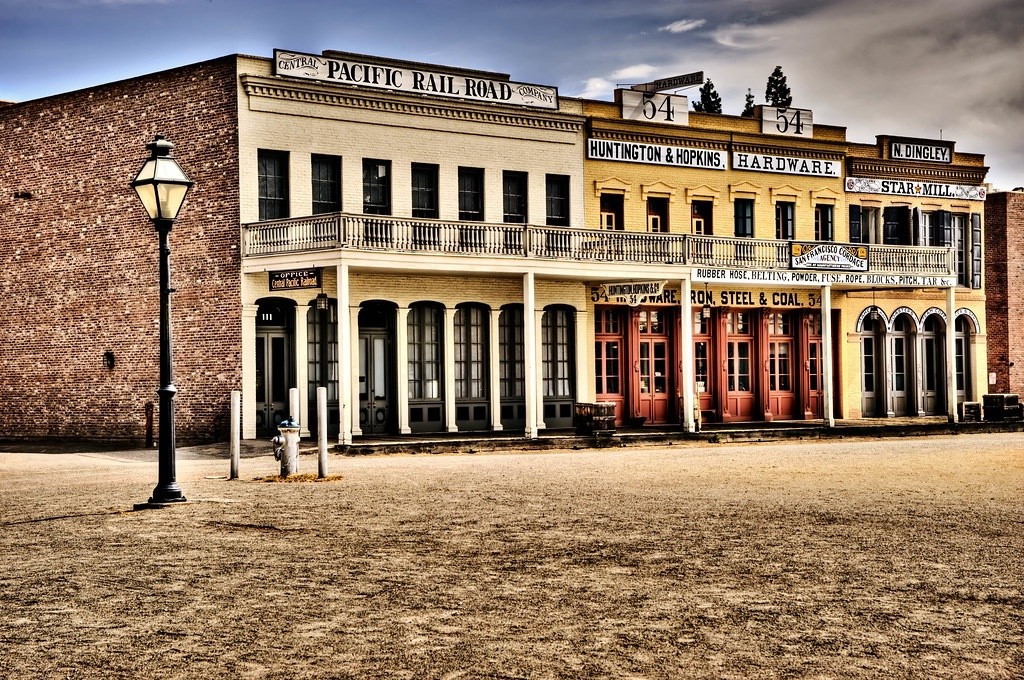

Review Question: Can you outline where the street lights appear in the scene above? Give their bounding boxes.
[127,133,196,509]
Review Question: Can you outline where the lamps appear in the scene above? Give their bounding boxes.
[317,269,328,311]
[103,349,115,370]
[703,282,710,320]
[871,288,879,320]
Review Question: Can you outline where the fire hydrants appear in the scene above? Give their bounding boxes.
[271,416,302,479]
